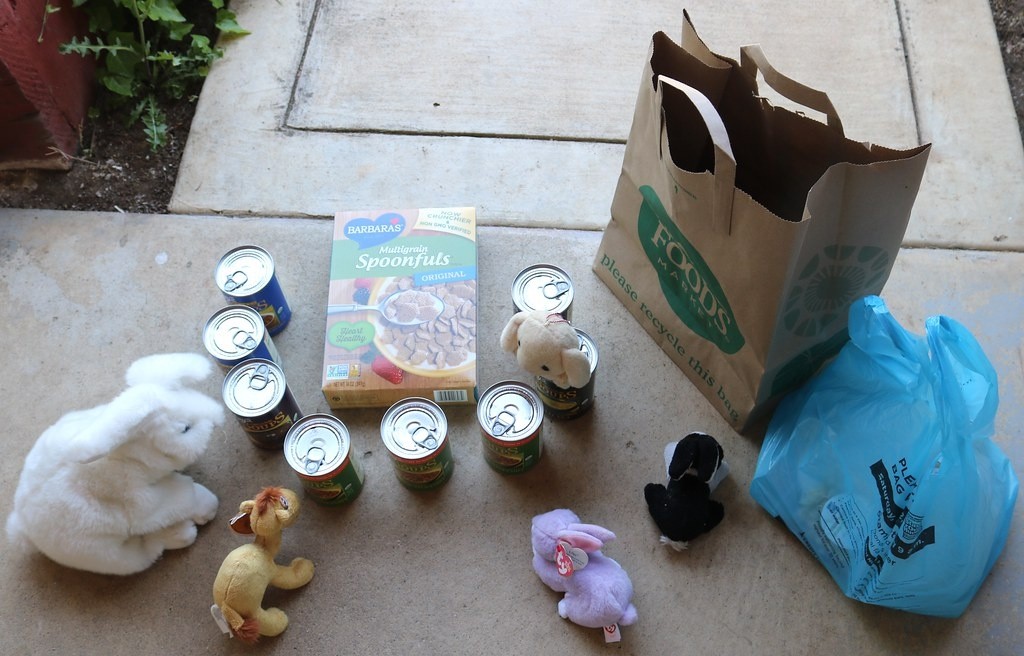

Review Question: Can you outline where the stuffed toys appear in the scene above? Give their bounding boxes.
[207,484,316,642]
[644,432,731,552]
[5,350,226,576]
[529,508,636,644]
[499,310,593,392]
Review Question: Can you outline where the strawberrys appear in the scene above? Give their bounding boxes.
[370,355,403,384]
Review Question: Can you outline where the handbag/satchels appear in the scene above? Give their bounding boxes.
[592,8,933,435]
[749,296,1019,620]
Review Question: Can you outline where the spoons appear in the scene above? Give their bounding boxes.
[327,290,444,325]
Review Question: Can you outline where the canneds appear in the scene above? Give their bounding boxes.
[476,379,545,475]
[203,305,283,376]
[380,396,454,490]
[511,263,574,325]
[283,413,365,507]
[222,358,303,453]
[214,244,292,337]
[533,328,599,420]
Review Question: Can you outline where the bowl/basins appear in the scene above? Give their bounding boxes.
[368,277,476,377]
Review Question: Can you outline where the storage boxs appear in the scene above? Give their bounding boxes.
[320,204,483,409]
